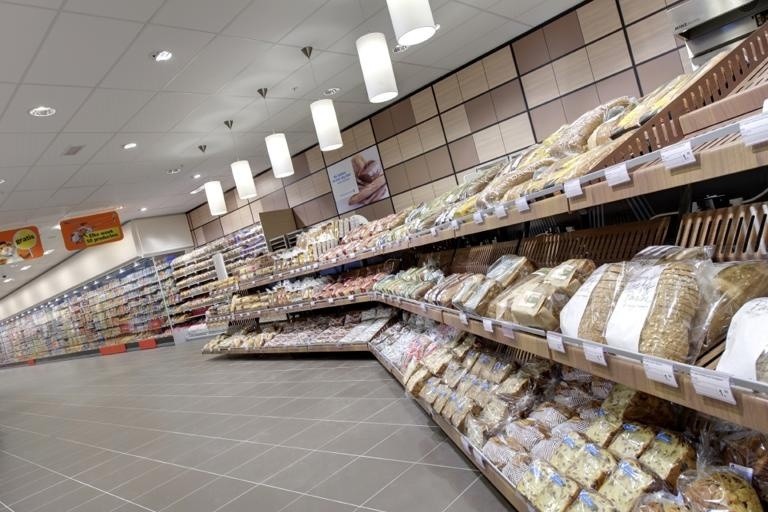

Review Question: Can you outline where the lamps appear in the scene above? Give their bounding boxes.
[386,0,436,47]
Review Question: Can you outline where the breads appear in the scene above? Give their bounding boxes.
[201,96,768,512]
[348,155,386,205]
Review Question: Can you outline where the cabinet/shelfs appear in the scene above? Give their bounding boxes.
[0,26,768,512]
